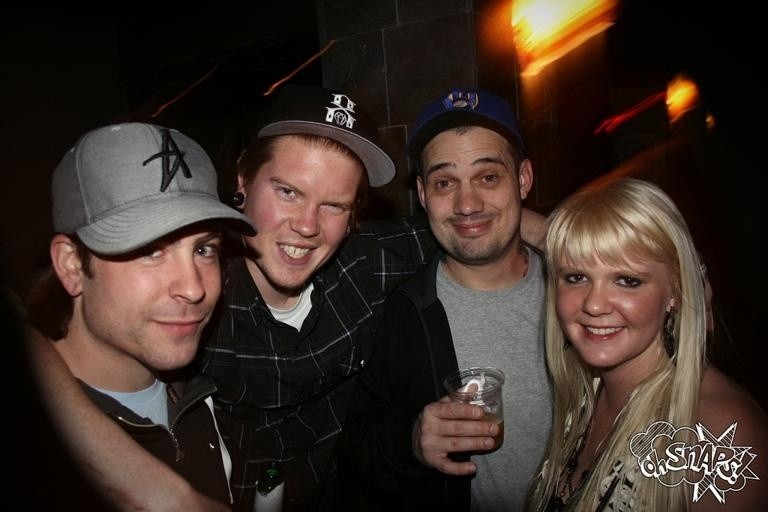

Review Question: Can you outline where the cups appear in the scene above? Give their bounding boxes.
[446,367,504,456]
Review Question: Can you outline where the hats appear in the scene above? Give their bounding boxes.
[253,84,397,188]
[51,122,258,257]
[404,86,524,162]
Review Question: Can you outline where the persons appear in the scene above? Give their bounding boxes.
[1,86,768,512]
